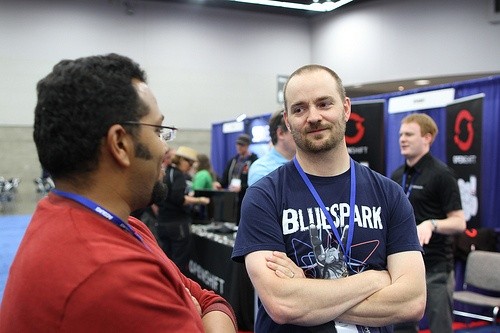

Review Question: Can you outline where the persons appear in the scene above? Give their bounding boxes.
[247,110,296,187]
[214,134,257,194]
[390,114,467,333]
[231,65,427,333]
[0,53,237,333]
[146,146,214,272]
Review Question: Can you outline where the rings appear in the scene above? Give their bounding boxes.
[291,274,294,278]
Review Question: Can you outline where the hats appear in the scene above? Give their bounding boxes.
[236,134,252,145]
[175,146,199,162]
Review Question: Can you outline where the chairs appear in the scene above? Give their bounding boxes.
[454,251,500,324]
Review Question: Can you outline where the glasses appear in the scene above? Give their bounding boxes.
[184,158,193,166]
[122,121,179,142]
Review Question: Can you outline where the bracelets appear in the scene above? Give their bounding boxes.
[431,219,437,232]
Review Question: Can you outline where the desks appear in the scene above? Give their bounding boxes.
[187,216,254,333]
